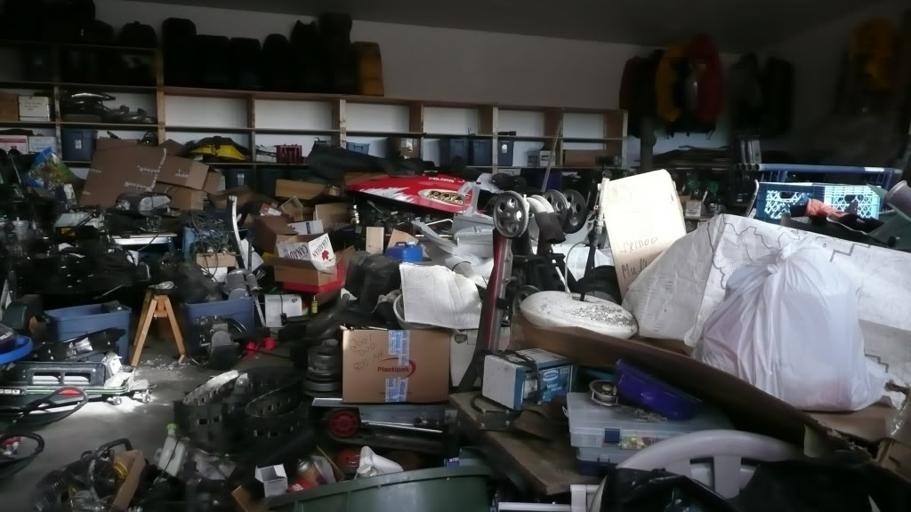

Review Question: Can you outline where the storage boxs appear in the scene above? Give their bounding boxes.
[437,137,515,169]
[315,139,372,157]
[0,90,105,168]
[182,293,257,359]
[43,301,132,369]
[153,151,363,296]
[340,321,454,405]
[562,148,615,168]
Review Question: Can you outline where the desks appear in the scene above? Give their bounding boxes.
[443,389,611,498]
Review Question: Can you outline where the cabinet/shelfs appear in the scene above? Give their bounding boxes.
[0,36,170,171]
[154,84,632,183]
